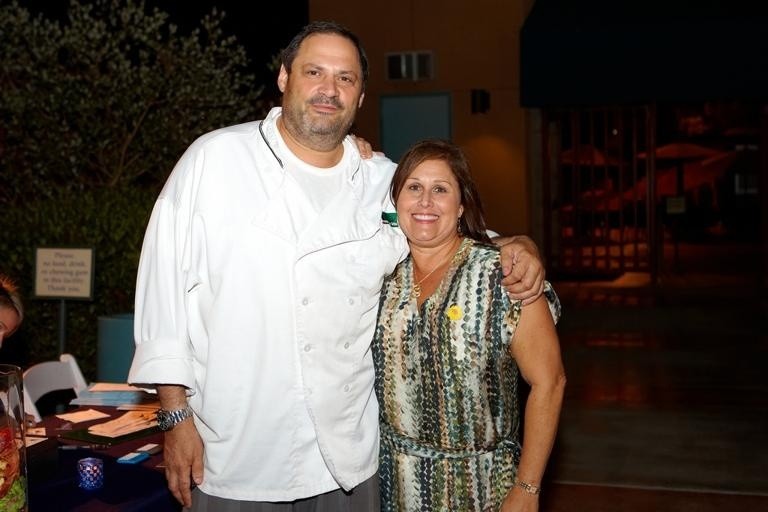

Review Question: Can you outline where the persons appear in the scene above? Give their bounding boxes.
[0,275,25,347]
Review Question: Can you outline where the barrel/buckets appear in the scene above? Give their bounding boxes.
[97,315,135,383]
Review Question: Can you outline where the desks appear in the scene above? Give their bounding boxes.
[18,400,183,510]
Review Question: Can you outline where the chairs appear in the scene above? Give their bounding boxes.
[0,351,87,427]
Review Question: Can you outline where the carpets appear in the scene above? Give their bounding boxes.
[549,401,768,496]
